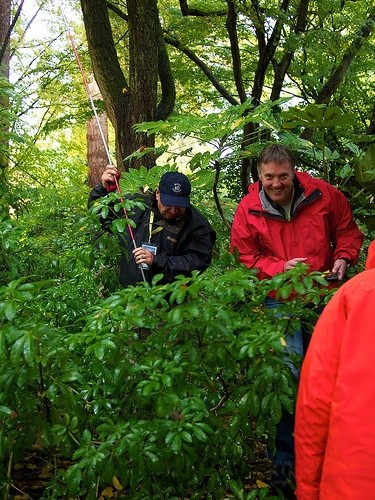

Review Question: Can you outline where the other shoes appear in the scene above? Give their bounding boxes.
[269,450,297,485]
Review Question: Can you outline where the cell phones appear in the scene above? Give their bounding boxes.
[324,274,337,281]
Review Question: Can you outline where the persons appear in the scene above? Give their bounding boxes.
[294,239,375,500]
[230,145,365,477]
[87,165,217,340]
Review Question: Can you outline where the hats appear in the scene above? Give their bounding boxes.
[160,172,191,208]
